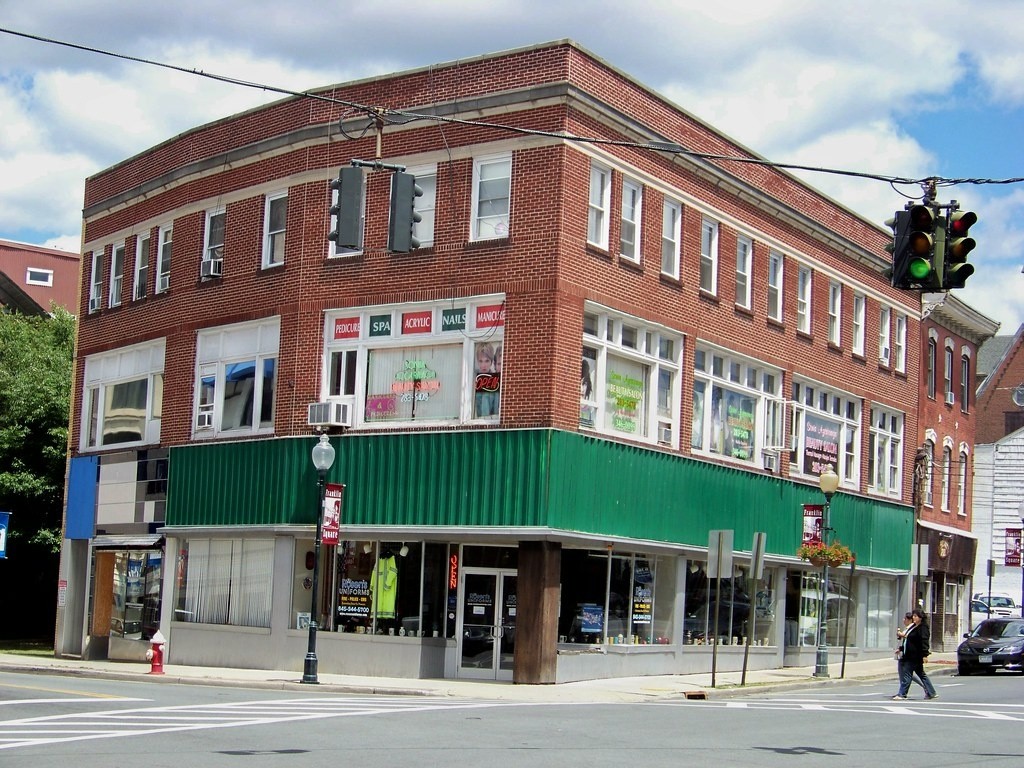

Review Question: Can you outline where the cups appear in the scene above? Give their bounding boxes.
[357,626,365,634]
[694,636,769,646]
[631,635,634,644]
[596,638,599,644]
[624,638,628,644]
[609,637,614,645]
[614,637,619,644]
[635,635,638,644]
[433,631,438,638]
[389,628,394,636]
[366,627,373,634]
[409,631,414,636]
[338,625,345,633]
[607,637,609,644]
[417,630,425,637]
[559,635,567,643]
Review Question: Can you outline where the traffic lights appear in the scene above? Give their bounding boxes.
[905,203,938,284]
[945,208,977,288]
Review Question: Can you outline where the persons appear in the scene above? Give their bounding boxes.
[893,612,927,697]
[892,609,939,700]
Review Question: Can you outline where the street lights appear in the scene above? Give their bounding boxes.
[297,432,337,682]
[812,463,839,677]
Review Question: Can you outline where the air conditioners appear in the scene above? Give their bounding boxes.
[763,456,776,470]
[307,401,353,428]
[90,298,102,311]
[160,276,170,291]
[200,258,223,277]
[878,346,889,360]
[921,490,932,504]
[658,427,672,445]
[945,391,955,405]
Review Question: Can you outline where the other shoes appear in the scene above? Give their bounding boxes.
[891,695,908,701]
[922,693,939,700]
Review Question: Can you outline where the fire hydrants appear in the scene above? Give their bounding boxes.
[144,628,168,675]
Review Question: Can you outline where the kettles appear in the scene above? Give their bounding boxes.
[399,627,405,636]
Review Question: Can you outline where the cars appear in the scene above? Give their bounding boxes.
[555,578,897,650]
[957,618,1024,676]
[969,590,1021,631]
